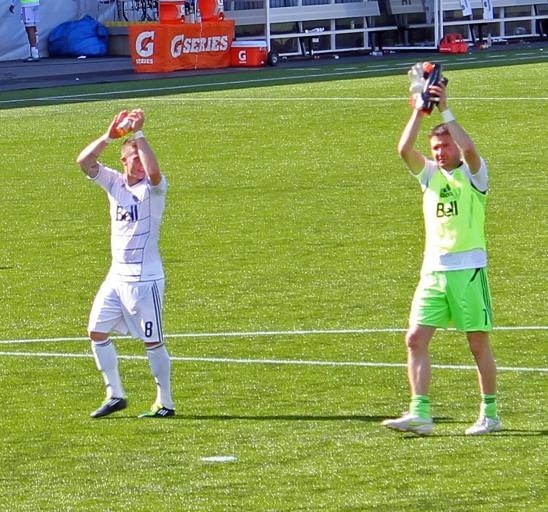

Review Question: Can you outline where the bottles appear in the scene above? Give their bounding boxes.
[420,63,448,115]
[179,5,201,23]
[479,34,493,52]
[115,109,144,137]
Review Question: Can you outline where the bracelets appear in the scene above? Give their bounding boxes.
[440,109,455,123]
[103,133,114,144]
[134,130,144,140]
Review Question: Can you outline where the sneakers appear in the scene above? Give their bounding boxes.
[23,55,41,62]
[381,412,433,436]
[464,415,502,436]
[90,396,128,418]
[138,403,175,418]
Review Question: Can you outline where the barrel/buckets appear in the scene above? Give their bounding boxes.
[159,0,185,24]
[197,0,218,21]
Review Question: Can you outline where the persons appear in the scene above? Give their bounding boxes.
[77,108,176,419]
[9,0,41,62]
[382,82,503,436]
